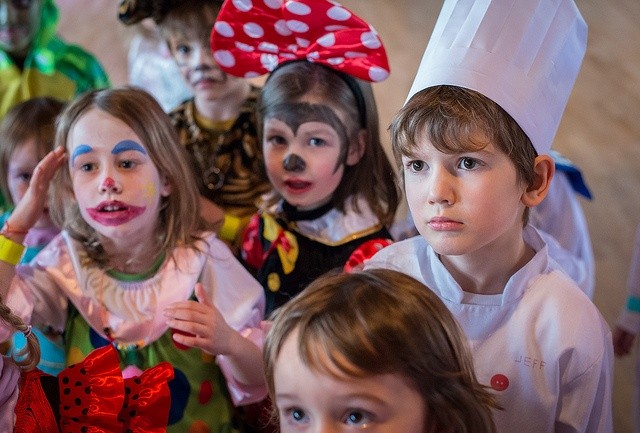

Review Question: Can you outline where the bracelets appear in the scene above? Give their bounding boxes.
[0,223,29,267]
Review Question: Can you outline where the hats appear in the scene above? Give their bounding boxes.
[402,0,589,154]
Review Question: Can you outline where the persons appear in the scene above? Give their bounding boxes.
[0,97,67,378]
[263,269,505,433]
[211,0,401,310]
[0,88,265,433]
[0,299,192,433]
[528,152,591,299]
[0,0,112,126]
[612,223,640,433]
[119,0,273,255]
[352,0,611,433]
